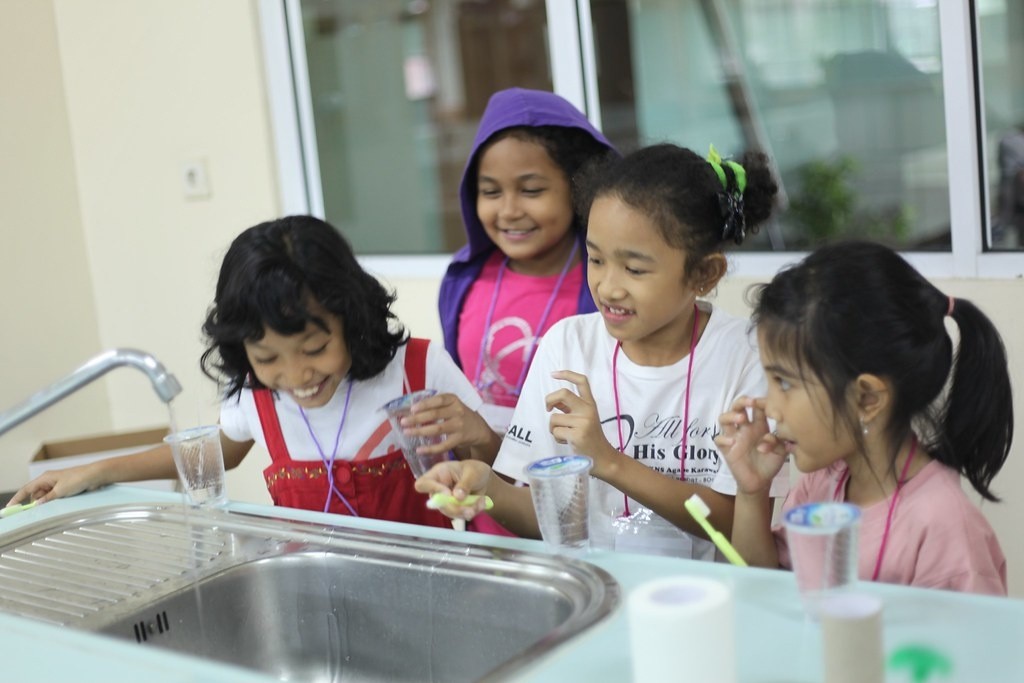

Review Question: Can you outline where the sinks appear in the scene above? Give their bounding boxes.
[89,520,622,683]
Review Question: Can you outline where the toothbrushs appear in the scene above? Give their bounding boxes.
[0,498,39,519]
[684,493,750,567]
[426,492,494,510]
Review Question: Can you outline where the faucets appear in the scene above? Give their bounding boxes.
[0,346,183,438]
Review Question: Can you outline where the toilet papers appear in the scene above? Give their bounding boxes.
[626,574,736,683]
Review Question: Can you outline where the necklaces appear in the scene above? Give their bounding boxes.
[833,435,916,581]
[612,307,698,517]
[298,380,359,517]
[475,242,576,395]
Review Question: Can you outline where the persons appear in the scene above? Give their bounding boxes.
[412,142,791,561]
[438,86,631,535]
[715,240,1015,597]
[7,214,510,535]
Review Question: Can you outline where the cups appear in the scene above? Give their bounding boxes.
[783,503,861,618]
[162,426,227,509]
[524,455,593,552]
[384,390,448,480]
